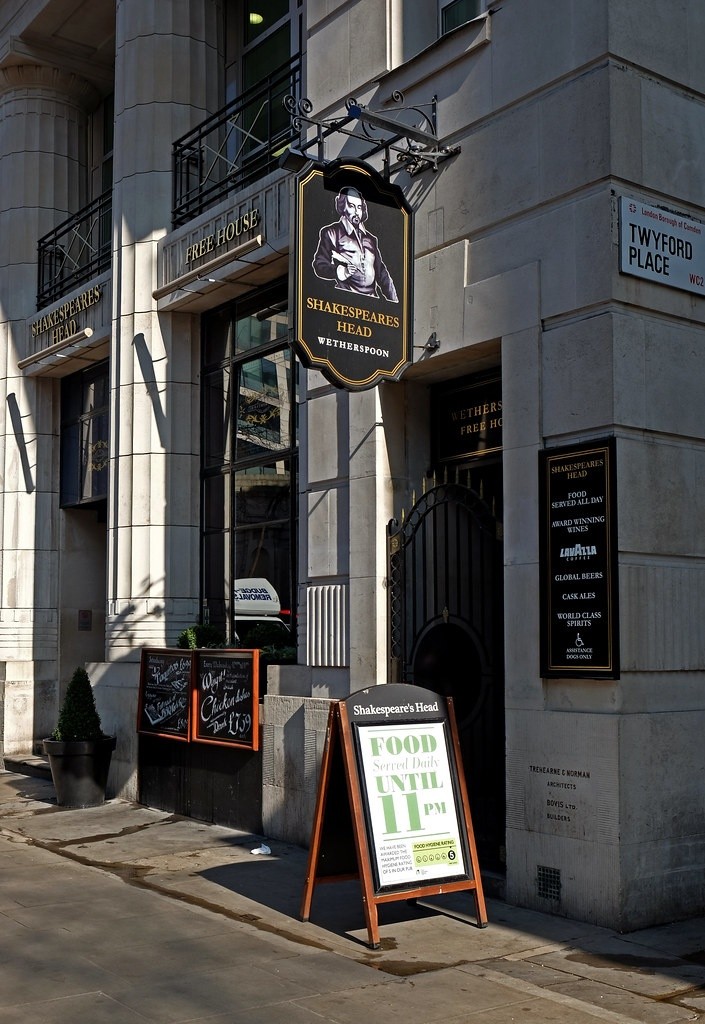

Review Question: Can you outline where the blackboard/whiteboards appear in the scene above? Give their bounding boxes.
[193,648,260,751]
[137,647,193,744]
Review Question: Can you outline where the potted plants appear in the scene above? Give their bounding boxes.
[43,665,116,808]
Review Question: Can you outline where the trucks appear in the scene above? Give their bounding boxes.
[234,578,291,648]
